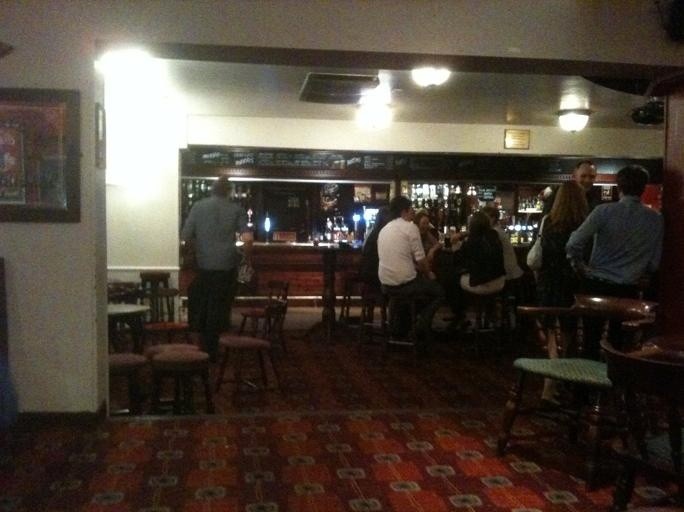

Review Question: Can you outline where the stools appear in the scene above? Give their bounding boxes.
[99,260,291,420]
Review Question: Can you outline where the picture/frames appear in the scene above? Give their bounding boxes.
[0,85,80,228]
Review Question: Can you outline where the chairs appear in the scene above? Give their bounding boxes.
[497,293,683,508]
[334,257,532,340]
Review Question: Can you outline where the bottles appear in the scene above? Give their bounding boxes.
[409,183,467,248]
[181,179,212,216]
[517,184,550,245]
[323,216,350,243]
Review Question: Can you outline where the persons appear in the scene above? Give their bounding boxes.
[181,178,254,351]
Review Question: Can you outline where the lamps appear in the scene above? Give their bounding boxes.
[552,93,595,135]
